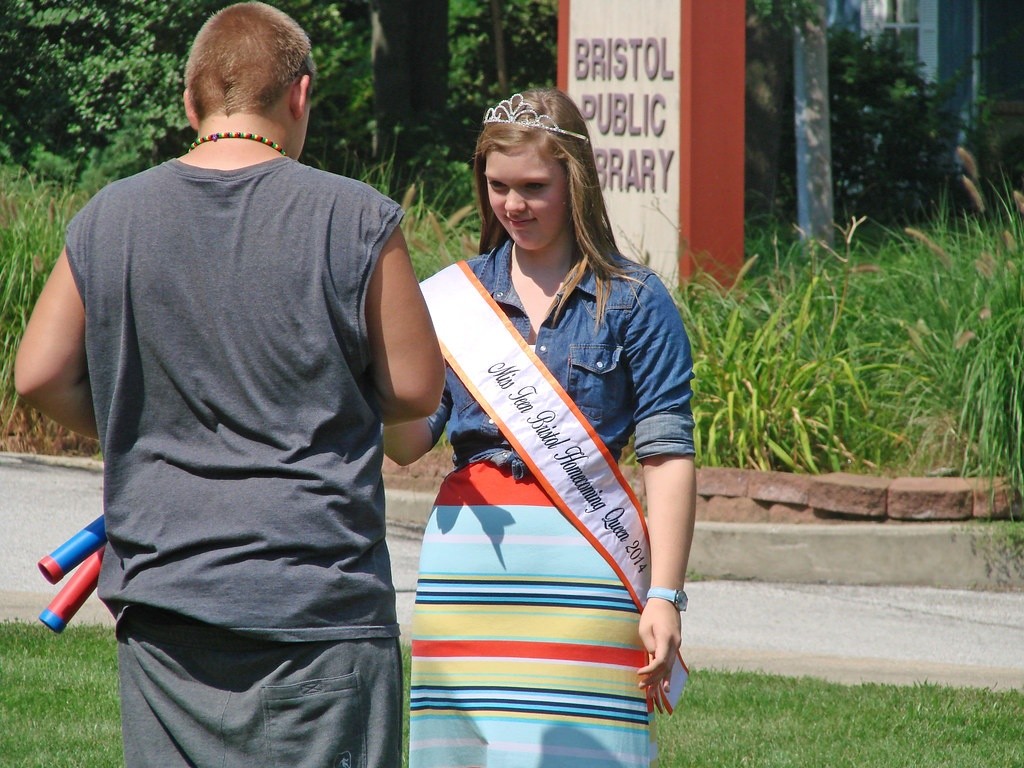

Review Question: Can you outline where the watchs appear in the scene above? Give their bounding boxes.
[646,587,688,611]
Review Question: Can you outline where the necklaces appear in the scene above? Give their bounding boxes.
[188,132,286,156]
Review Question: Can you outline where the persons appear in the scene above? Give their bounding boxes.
[382,87,696,768]
[14,1,445,768]
